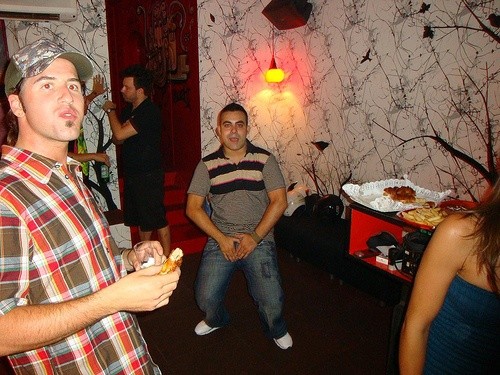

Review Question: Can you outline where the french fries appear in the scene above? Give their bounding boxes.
[402,206,446,227]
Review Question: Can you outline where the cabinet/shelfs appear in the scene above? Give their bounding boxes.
[341,191,479,288]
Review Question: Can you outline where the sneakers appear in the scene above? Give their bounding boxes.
[273,331,293,350]
[194,320,220,335]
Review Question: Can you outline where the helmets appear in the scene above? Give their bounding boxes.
[283,186,344,223]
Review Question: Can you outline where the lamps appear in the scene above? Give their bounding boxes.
[265,26,285,82]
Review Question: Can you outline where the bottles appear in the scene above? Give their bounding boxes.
[99,151,110,184]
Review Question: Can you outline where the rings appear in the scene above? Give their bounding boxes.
[106,159,107,161]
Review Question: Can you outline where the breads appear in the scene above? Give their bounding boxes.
[159,248,183,275]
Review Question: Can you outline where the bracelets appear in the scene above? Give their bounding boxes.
[250,231,263,245]
[127,248,134,266]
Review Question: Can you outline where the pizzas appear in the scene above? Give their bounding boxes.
[384,186,416,204]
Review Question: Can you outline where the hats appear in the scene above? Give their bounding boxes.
[5,38,93,98]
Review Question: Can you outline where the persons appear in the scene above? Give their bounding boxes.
[185,102,295,351]
[67,73,112,192]
[0,37,183,375]
[101,64,172,258]
[397,178,500,375]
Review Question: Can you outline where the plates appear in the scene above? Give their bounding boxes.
[438,199,479,216]
[396,207,436,230]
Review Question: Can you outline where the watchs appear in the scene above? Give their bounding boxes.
[107,108,116,114]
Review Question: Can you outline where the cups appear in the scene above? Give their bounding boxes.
[134,241,155,270]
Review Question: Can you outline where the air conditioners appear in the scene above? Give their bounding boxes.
[0,0,78,20]
